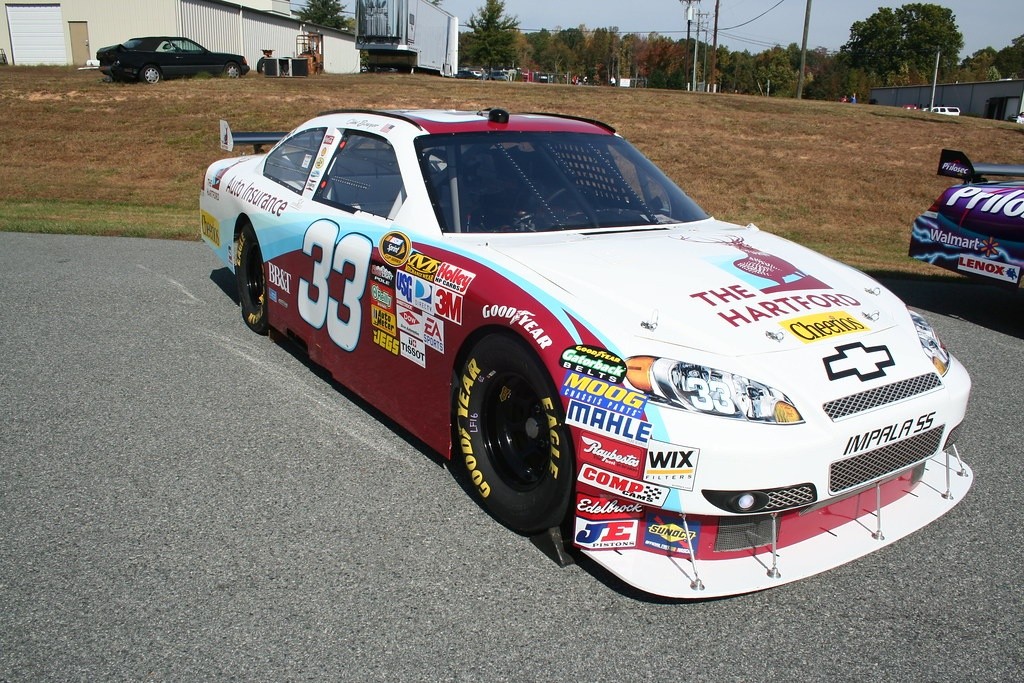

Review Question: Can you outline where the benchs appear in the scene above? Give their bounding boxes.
[275,147,404,218]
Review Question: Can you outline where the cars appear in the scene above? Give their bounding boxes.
[96,36,250,86]
[902,103,918,110]
[457,64,556,84]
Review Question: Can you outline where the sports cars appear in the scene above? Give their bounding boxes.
[199,107,974,599]
[908,148,1024,289]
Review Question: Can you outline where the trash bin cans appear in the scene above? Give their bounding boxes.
[0,48,8,65]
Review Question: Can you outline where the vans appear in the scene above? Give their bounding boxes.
[930,107,961,116]
